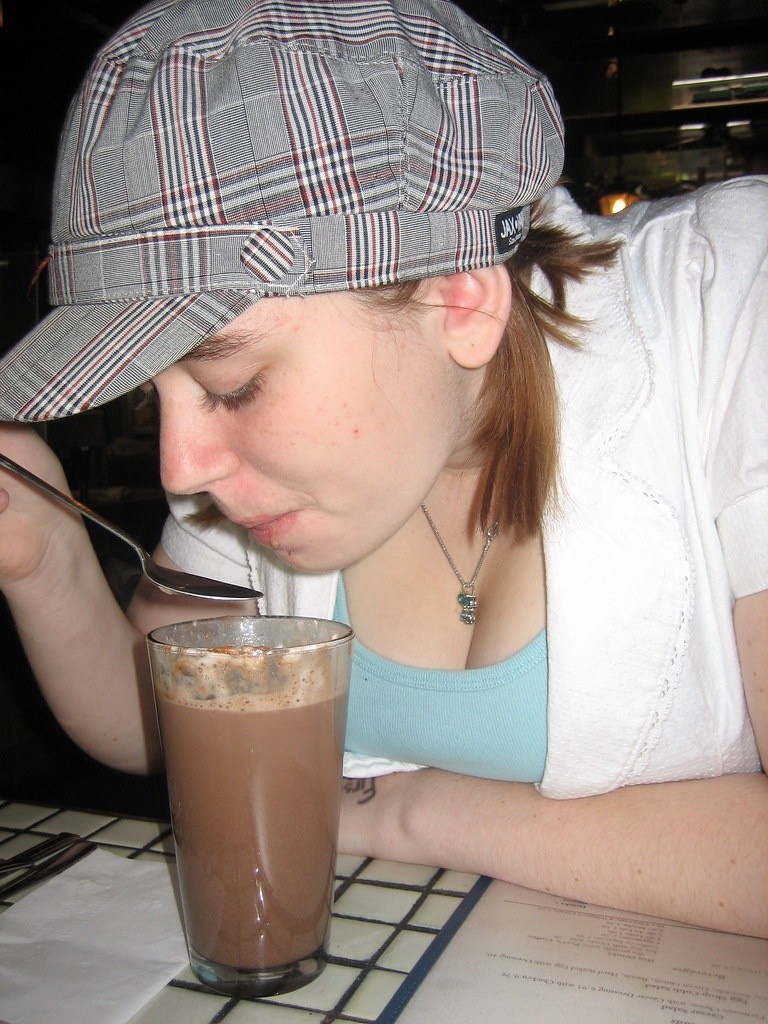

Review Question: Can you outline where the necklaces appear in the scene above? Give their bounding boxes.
[420,502,500,626]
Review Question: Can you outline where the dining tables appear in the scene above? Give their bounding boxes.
[0,800,768,1024]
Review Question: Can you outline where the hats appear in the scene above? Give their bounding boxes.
[0,0,566,424]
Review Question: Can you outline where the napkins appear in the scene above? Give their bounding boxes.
[0,847,191,1024]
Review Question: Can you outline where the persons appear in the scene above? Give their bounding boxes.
[0,0,768,942]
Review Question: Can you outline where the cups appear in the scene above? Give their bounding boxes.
[144,614,356,994]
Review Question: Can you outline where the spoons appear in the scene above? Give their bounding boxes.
[0,452,264,603]
